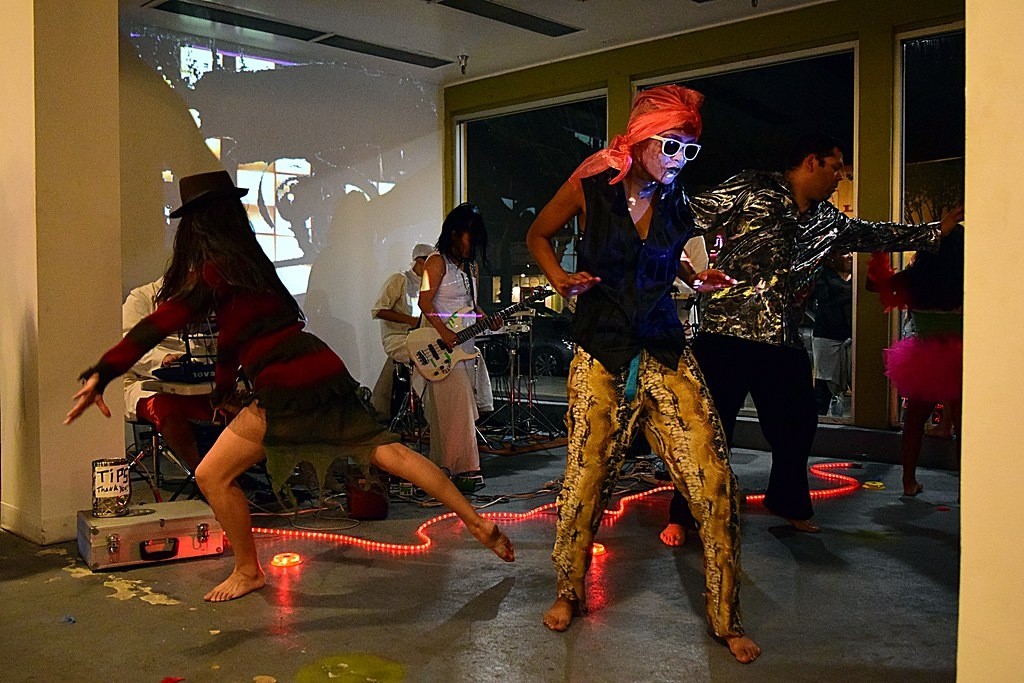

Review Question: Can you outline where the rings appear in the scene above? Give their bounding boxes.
[453,342,456,344]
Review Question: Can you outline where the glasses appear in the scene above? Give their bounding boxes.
[651,134,703,161]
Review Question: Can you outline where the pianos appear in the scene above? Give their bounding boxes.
[150,360,215,385]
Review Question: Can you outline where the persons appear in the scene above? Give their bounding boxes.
[667,135,962,536]
[417,202,504,477]
[865,224,963,496]
[63,169,516,601]
[121,262,219,472]
[370,243,437,364]
[526,84,761,663]
[807,258,853,415]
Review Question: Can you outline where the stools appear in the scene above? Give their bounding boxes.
[126,418,202,484]
[389,359,428,438]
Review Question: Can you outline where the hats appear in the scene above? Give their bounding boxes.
[411,243,437,261]
[169,170,249,219]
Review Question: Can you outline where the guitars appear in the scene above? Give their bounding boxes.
[404,289,557,383]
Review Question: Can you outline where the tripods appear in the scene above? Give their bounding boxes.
[476,320,565,451]
